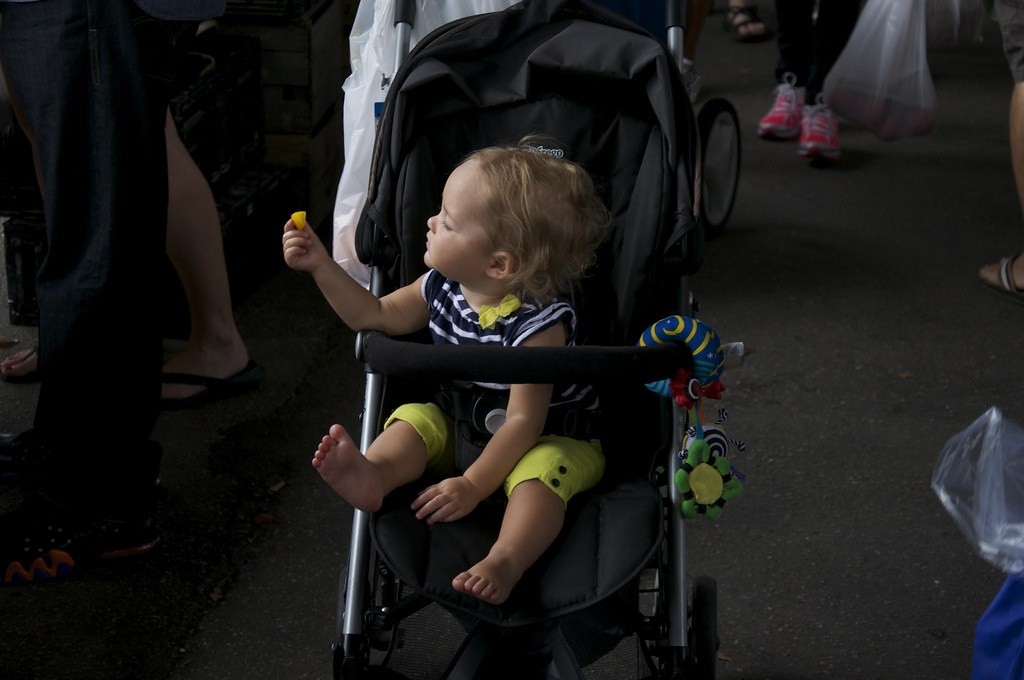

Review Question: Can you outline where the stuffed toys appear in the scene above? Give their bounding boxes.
[637,315,747,519]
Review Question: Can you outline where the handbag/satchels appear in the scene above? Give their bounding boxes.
[821,0,939,141]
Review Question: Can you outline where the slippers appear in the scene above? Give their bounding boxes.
[161,358,266,410]
[0,345,42,383]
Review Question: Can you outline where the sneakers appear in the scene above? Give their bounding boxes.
[0,485,163,586]
[757,71,809,139]
[797,93,846,165]
[0,428,164,492]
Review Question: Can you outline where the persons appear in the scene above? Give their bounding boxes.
[283,147,605,605]
[757,0,862,164]
[1,0,249,587]
[974,0,1024,310]
[685,0,768,108]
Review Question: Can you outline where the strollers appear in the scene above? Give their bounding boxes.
[332,0,722,680]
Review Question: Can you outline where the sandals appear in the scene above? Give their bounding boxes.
[722,5,769,43]
[977,252,1024,305]
